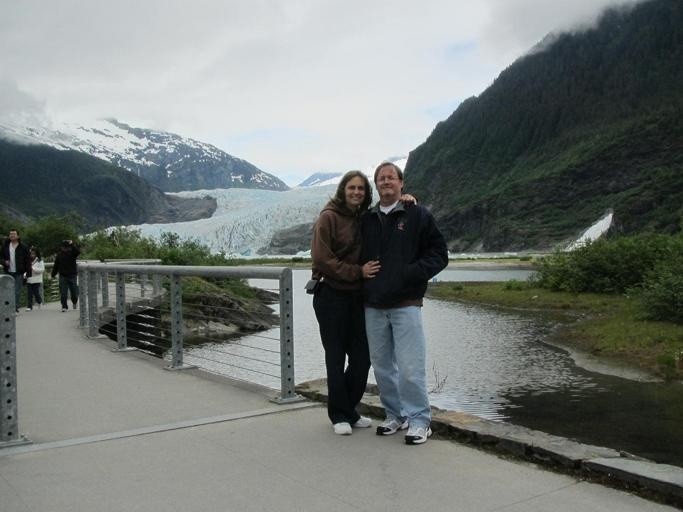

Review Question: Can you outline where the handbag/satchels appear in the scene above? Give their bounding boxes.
[303,280,319,294]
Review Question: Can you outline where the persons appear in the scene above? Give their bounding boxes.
[0,226,33,316]
[358,160,453,446]
[49,238,82,312]
[20,244,43,313]
[308,170,422,436]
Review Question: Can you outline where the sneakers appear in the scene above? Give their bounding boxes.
[351,418,370,428]
[376,419,408,436]
[334,422,352,434]
[404,427,432,444]
[73,303,76,309]
[62,308,66,312]
[25,308,30,311]
